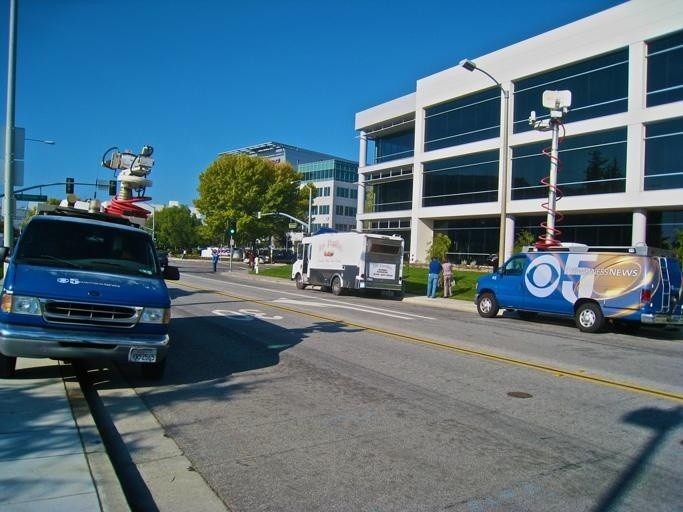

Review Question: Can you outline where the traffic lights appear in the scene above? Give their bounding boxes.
[230,219,236,235]
[110,181,116,195]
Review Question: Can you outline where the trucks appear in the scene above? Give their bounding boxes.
[291,232,405,296]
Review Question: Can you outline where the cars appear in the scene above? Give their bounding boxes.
[258,250,297,264]
[0,207,180,381]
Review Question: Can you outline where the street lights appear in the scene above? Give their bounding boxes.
[290,180,312,232]
[24,139,55,145]
[459,58,509,267]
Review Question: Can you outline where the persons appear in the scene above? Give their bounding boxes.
[211,249,219,272]
[249,252,259,274]
[182,248,187,260]
[488,255,499,273]
[427,257,456,298]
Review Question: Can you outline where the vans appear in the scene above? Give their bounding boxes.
[474,242,683,333]
